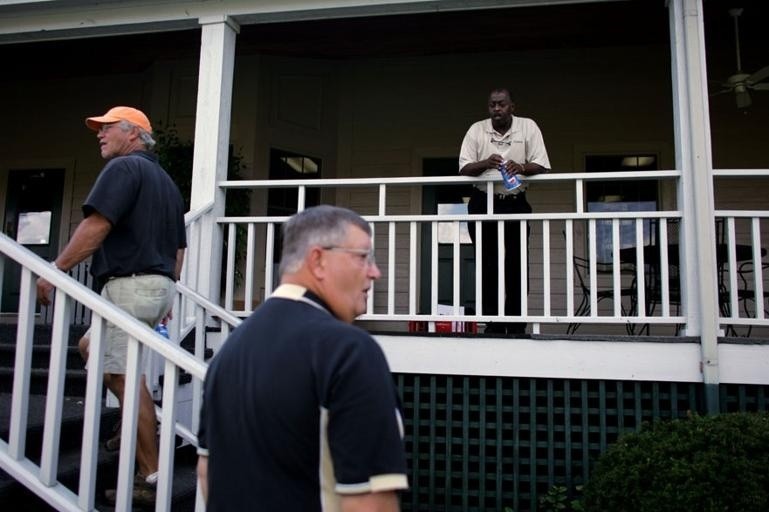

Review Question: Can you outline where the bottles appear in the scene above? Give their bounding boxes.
[156,319,168,340]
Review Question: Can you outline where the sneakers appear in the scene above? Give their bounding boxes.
[484,326,506,334]
[103,473,157,507]
[105,421,120,454]
[507,327,526,335]
[105,268,181,283]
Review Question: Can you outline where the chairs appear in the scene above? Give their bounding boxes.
[561,217,769,337]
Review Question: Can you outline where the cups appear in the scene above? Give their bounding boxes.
[499,161,523,191]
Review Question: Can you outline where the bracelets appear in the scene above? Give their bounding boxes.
[520,164,525,174]
[51,261,57,268]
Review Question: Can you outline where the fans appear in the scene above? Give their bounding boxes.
[709,6,769,111]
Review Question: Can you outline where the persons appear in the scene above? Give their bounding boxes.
[36,104,188,504]
[459,87,552,335]
[197,204,410,512]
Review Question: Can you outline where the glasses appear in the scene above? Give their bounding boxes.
[321,244,375,262]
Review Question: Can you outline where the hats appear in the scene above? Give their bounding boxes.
[85,106,154,134]
